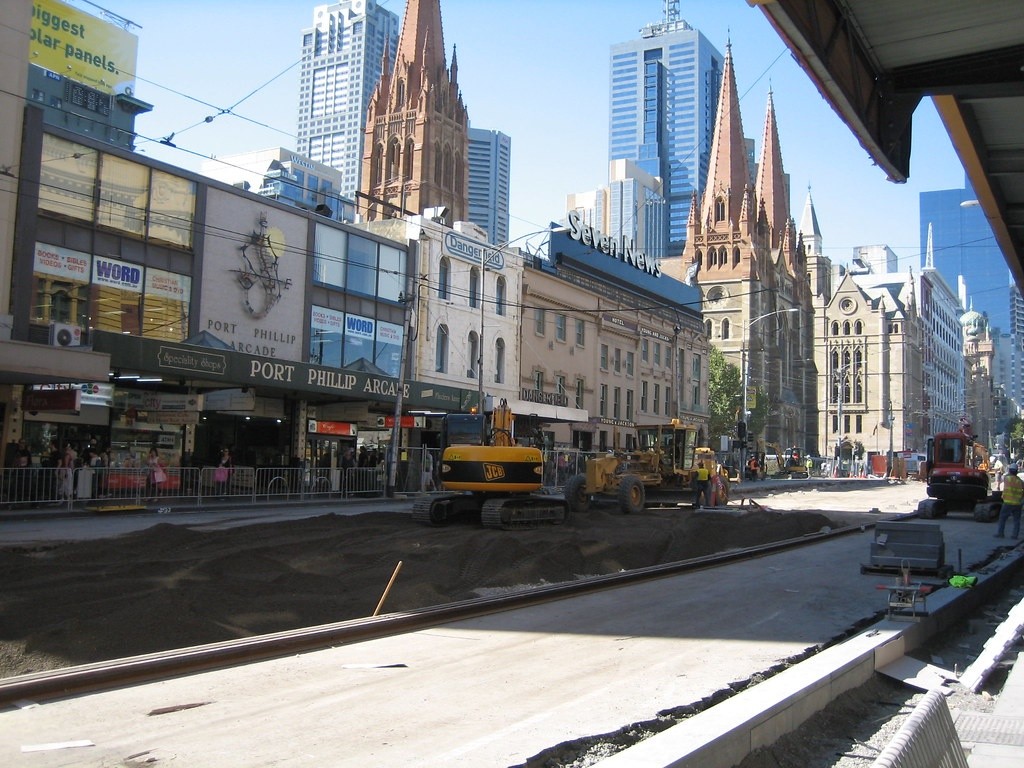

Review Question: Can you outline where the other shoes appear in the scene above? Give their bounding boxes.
[143,496,153,502]
[106,493,113,497]
[98,493,106,498]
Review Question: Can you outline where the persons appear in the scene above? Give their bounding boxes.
[783,455,813,479]
[515,439,634,489]
[993,457,1004,491]
[319,446,440,497]
[691,463,713,508]
[747,454,760,482]
[5,438,164,503]
[992,463,1024,539]
[215,448,232,501]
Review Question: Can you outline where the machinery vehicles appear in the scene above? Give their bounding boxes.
[563,418,741,517]
[409,397,571,530]
[745,438,809,480]
[917,429,1003,523]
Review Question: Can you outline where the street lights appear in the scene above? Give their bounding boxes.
[740,308,799,481]
[477,226,574,414]
[837,360,869,477]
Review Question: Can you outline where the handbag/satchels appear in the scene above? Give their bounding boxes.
[213,464,229,482]
[150,464,168,483]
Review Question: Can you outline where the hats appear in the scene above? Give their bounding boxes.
[697,463,704,467]
[806,455,811,458]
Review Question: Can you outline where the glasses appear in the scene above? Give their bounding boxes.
[19,441,26,445]
[224,450,230,453]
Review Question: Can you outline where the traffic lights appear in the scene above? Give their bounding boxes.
[733,440,741,449]
[738,422,746,438]
[747,432,754,442]
[740,441,748,449]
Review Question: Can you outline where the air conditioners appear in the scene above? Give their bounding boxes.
[48,323,81,347]
